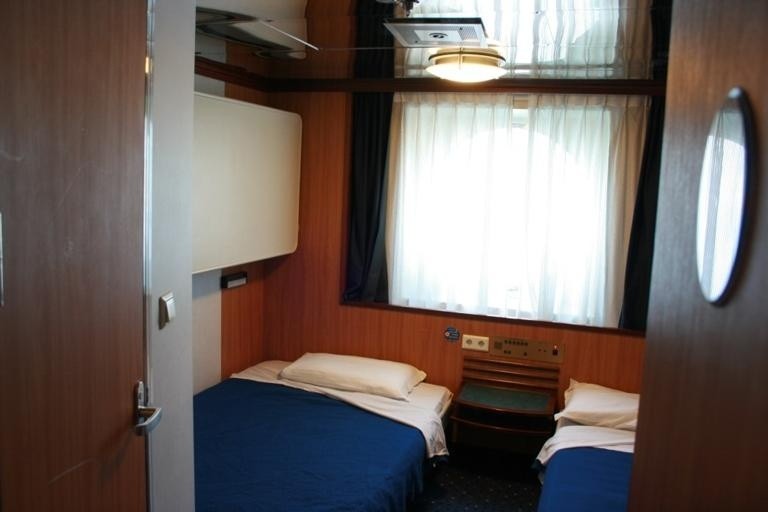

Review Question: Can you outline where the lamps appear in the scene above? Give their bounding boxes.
[425,47,509,87]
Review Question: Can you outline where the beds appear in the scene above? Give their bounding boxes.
[538,408,637,511]
[195,359,452,510]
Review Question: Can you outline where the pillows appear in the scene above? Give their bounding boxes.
[280,351,430,402]
[554,378,641,431]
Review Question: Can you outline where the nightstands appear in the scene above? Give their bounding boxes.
[450,379,559,480]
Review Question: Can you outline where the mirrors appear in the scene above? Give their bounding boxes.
[693,84,760,305]
